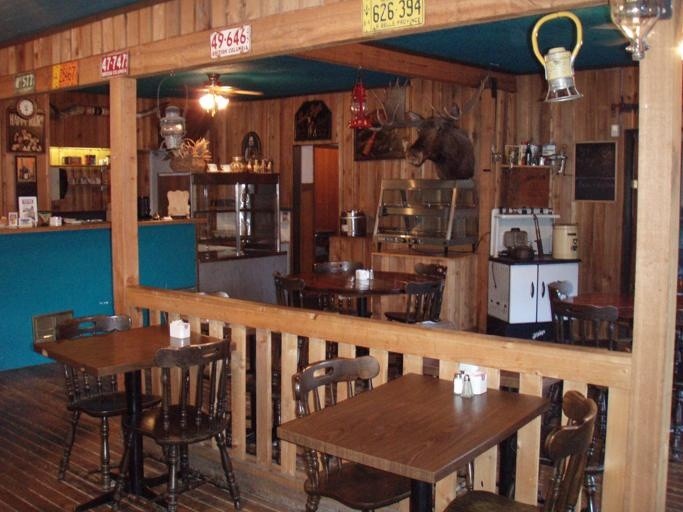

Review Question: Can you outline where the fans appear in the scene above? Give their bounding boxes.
[167,83,264,97]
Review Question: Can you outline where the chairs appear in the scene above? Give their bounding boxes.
[290,356,411,512]
[312,262,363,314]
[272,271,305,309]
[53,314,162,492]
[548,287,618,352]
[441,389,598,512]
[414,263,448,280]
[120,338,241,512]
[384,280,440,324]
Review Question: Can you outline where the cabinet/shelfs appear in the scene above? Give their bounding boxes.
[487,256,582,324]
[148,150,280,260]
[370,176,480,255]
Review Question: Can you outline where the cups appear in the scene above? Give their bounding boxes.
[39,212,53,224]
[220,165,232,170]
[208,164,218,170]
[49,216,63,226]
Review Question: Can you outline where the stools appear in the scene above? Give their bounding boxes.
[506,435,606,512]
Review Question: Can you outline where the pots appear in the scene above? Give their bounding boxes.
[340,208,367,238]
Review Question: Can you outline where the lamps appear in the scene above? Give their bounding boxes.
[531,12,584,104]
[608,0,661,62]
[155,74,189,150]
[198,92,229,121]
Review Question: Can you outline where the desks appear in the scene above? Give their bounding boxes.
[276,372,551,512]
[33,326,222,512]
[564,291,636,321]
[284,270,438,296]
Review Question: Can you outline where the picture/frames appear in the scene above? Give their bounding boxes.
[5,106,46,154]
[18,197,38,225]
[241,131,262,165]
[16,154,38,211]
[572,140,618,203]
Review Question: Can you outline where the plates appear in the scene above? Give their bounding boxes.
[220,170,231,173]
[206,170,220,173]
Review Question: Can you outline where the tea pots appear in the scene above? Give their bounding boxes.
[514,246,536,260]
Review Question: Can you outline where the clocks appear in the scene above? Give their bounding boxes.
[15,96,38,121]
